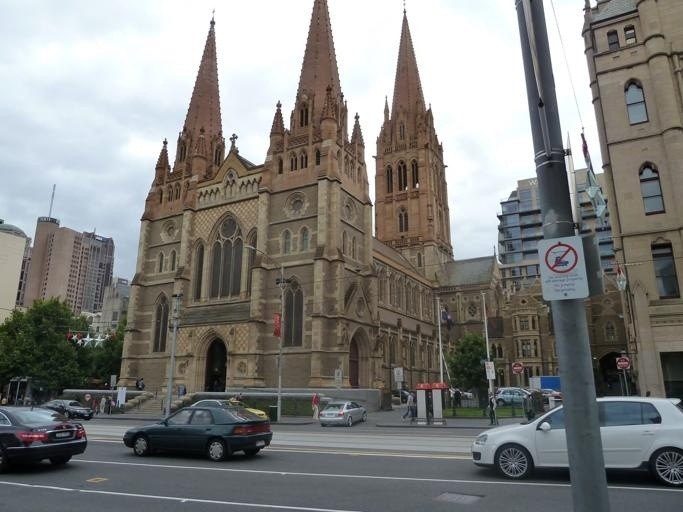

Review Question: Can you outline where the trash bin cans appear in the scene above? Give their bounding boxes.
[269,406,277,421]
[178,385,186,398]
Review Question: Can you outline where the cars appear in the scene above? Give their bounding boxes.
[448,386,563,407]
[188,398,269,425]
[319,400,366,428]
[0,405,87,472]
[41,399,93,420]
[122,407,273,463]
[392,389,431,402]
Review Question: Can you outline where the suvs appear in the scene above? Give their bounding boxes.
[469,396,681,488]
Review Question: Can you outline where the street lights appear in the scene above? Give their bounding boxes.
[165,292,182,417]
[242,243,286,424]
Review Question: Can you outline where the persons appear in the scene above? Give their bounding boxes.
[310,390,321,419]
[521,394,535,422]
[208,367,226,392]
[400,390,414,422]
[228,391,242,407]
[454,387,462,408]
[486,391,498,426]
[0,377,145,417]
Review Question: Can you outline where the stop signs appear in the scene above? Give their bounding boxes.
[616,357,630,370]
[511,362,524,375]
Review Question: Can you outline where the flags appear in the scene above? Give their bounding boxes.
[444,305,454,331]
